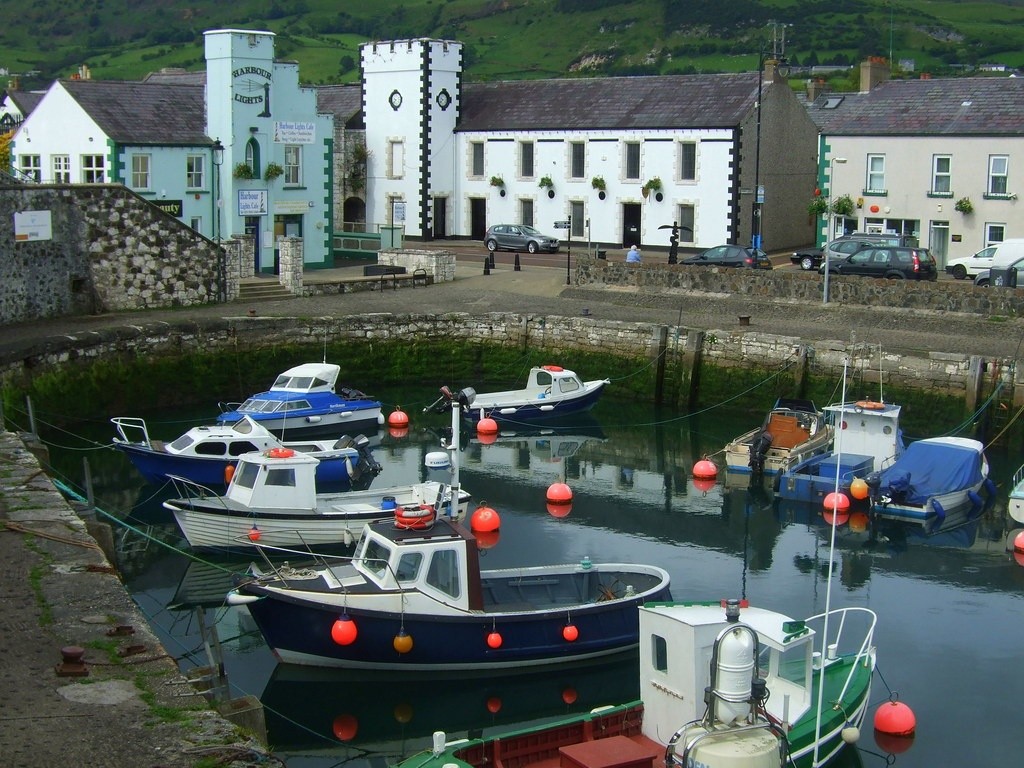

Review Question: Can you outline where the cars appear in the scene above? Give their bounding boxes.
[790,231,938,283]
[973,256,1024,290]
[678,244,773,270]
[945,238,1002,280]
[483,223,560,254]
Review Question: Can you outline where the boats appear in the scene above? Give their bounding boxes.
[165,547,378,653]
[161,390,474,556]
[387,359,880,768]
[214,324,387,441]
[723,393,828,477]
[869,434,991,526]
[259,643,640,768]
[108,399,385,503]
[224,385,676,672]
[421,364,611,428]
[776,341,907,507]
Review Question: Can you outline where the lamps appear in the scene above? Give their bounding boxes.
[27,138,30,142]
[249,127,258,133]
[89,137,94,141]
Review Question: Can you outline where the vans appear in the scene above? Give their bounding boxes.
[990,238,1024,272]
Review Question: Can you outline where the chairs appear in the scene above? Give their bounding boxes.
[876,253,883,262]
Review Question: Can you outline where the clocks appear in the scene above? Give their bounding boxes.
[391,93,401,107]
[438,94,447,106]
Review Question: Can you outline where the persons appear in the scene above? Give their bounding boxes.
[626,245,641,262]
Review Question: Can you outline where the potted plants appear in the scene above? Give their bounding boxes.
[955,197,973,214]
[491,177,502,188]
[832,194,853,217]
[809,198,828,216]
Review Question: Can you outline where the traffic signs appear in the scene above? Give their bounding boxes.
[553,221,571,229]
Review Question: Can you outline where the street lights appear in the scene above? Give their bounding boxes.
[821,157,848,304]
[211,136,225,304]
